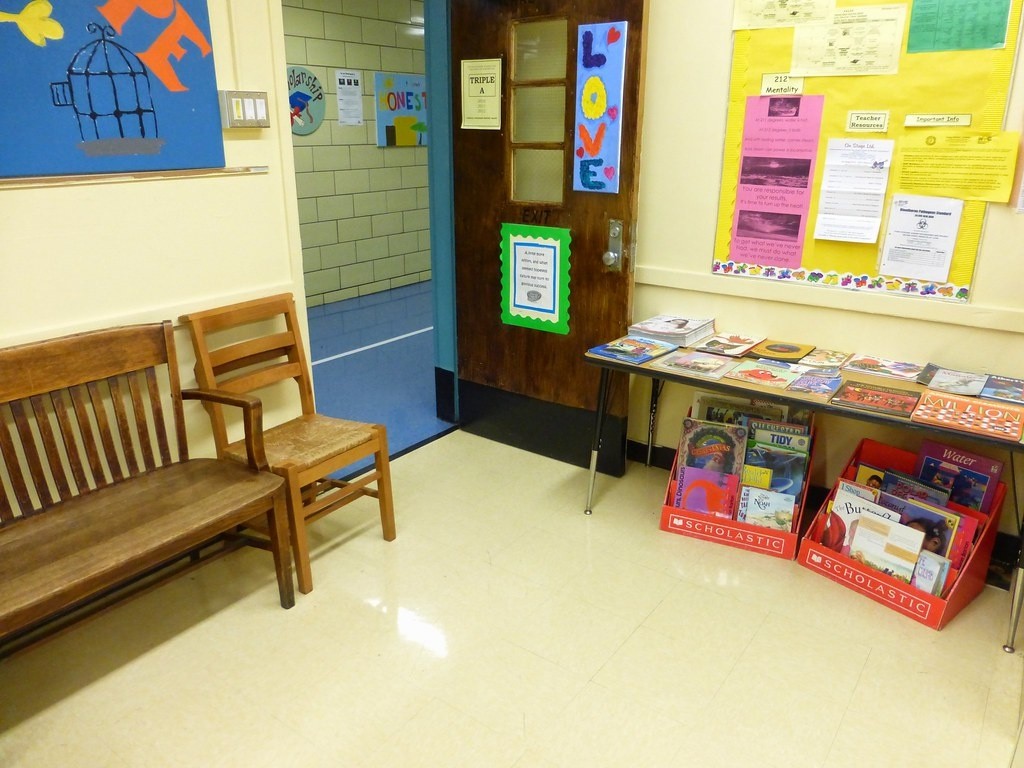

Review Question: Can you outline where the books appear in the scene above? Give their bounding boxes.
[586,315,1024,446]
[820,437,1005,600]
[671,391,814,536]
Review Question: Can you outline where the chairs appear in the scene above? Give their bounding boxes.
[178,293,397,594]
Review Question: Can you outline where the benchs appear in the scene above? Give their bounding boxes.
[0,318,294,643]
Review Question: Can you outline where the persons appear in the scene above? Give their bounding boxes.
[905,514,949,559]
[839,519,863,557]
[643,318,690,333]
[866,474,883,491]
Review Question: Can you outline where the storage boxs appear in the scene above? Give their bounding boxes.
[796,438,1010,632]
[659,390,820,561]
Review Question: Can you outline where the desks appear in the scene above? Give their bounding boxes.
[582,315,1024,653]
